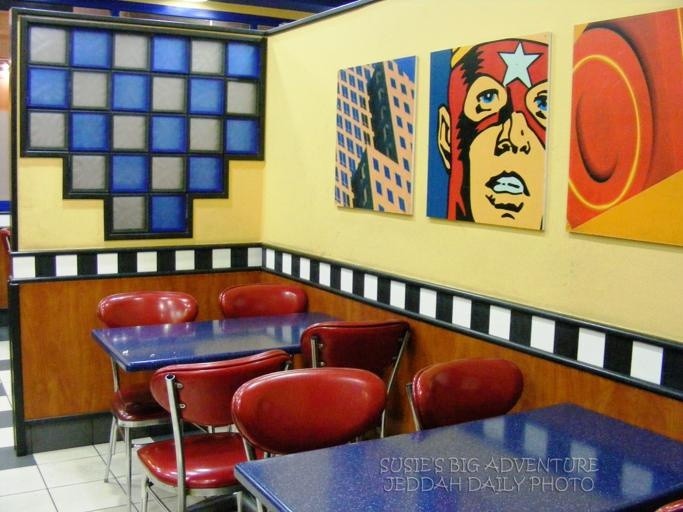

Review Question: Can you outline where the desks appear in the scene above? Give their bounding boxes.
[229,401,683,511]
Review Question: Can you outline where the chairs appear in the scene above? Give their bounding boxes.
[92,283,525,511]
